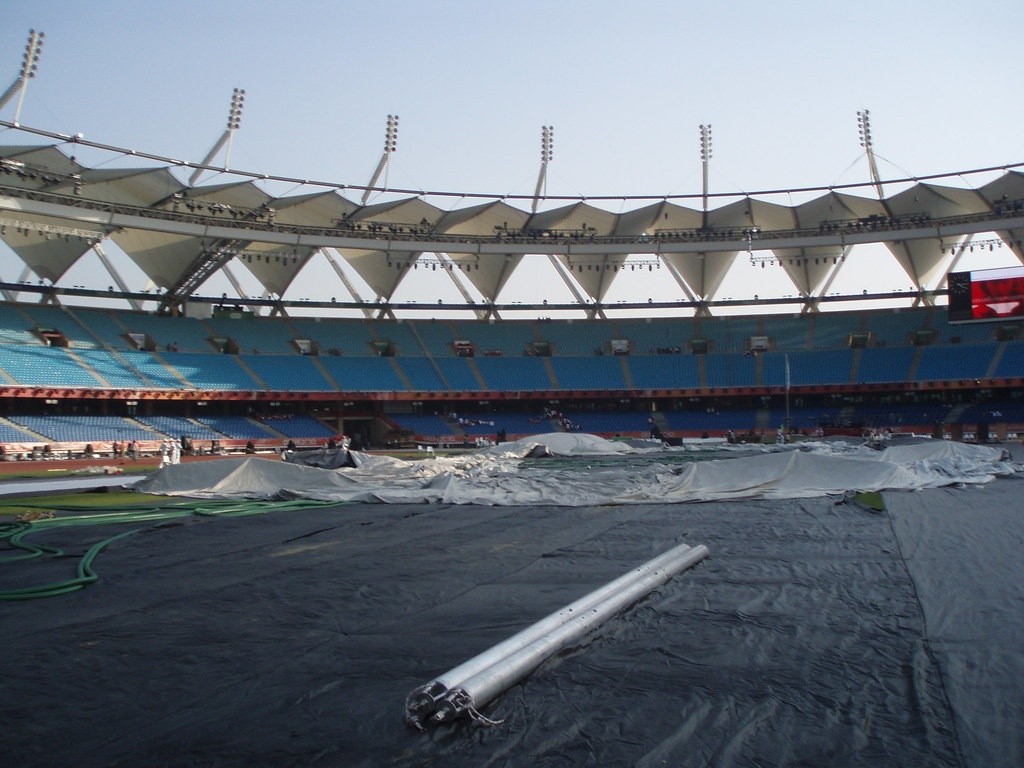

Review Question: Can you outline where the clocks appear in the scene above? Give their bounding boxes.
[949,275,971,295]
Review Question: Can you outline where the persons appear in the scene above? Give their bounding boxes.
[323,436,348,451]
[861,424,900,447]
[288,440,296,450]
[256,411,294,421]
[85,445,93,459]
[706,407,715,413]
[647,417,661,439]
[934,420,945,438]
[32,445,51,459]
[720,429,765,443]
[776,428,790,442]
[158,436,193,468]
[0,446,6,459]
[166,341,178,351]
[434,411,495,426]
[113,440,139,460]
[657,346,681,354]
[68,451,73,459]
[246,442,254,454]
[810,429,824,439]
[464,428,505,447]
[527,406,580,431]
[457,349,503,357]
